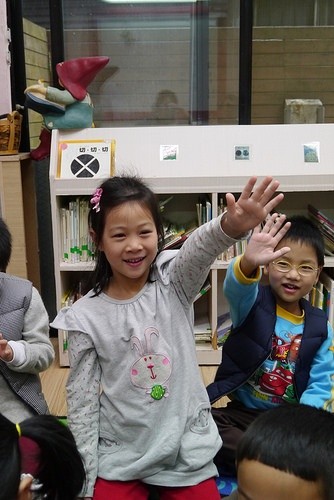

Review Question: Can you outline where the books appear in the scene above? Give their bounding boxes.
[196,201,256,260]
[308,205,334,257]
[62,292,87,352]
[302,273,334,328]
[61,197,96,263]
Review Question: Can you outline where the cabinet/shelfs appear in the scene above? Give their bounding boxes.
[50,123,334,370]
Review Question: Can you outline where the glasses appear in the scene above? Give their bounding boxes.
[272,261,318,277]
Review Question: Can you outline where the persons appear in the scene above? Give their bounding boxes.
[50,173,284,500]
[0,214,55,474]
[206,211,334,478]
[223,404,334,500]
[151,89,178,126]
[0,409,87,500]
